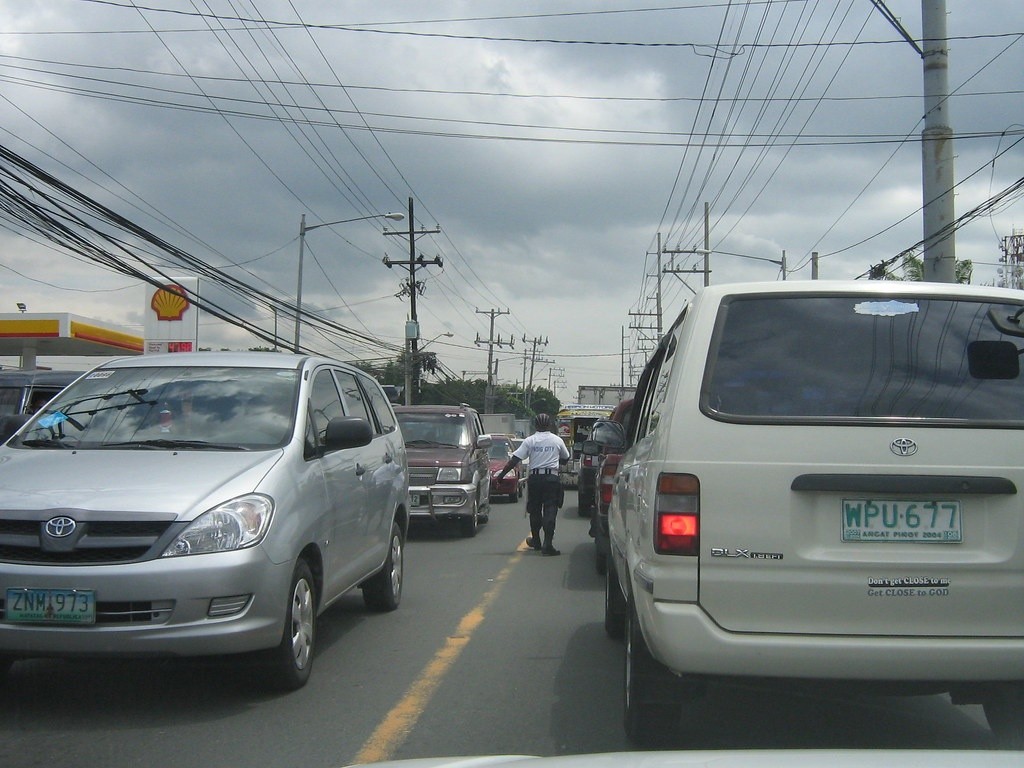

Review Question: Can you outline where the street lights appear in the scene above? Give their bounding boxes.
[403,332,456,404]
[693,248,787,281]
[293,210,405,352]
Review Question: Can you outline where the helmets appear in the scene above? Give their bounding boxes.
[535,413,550,431]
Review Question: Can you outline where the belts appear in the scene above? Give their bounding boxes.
[529,468,559,476]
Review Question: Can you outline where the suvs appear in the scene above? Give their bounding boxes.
[0,348,411,694]
[385,403,494,540]
[584,277,1024,753]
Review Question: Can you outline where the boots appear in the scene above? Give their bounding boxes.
[526,531,541,550]
[542,533,560,555]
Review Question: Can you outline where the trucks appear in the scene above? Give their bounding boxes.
[578,385,637,410]
[478,412,531,440]
[556,404,616,489]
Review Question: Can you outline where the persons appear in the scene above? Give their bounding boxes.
[498,414,570,555]
[27,395,51,415]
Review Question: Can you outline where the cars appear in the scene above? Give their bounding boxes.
[578,396,642,577]
[509,438,530,485]
[485,433,525,505]
